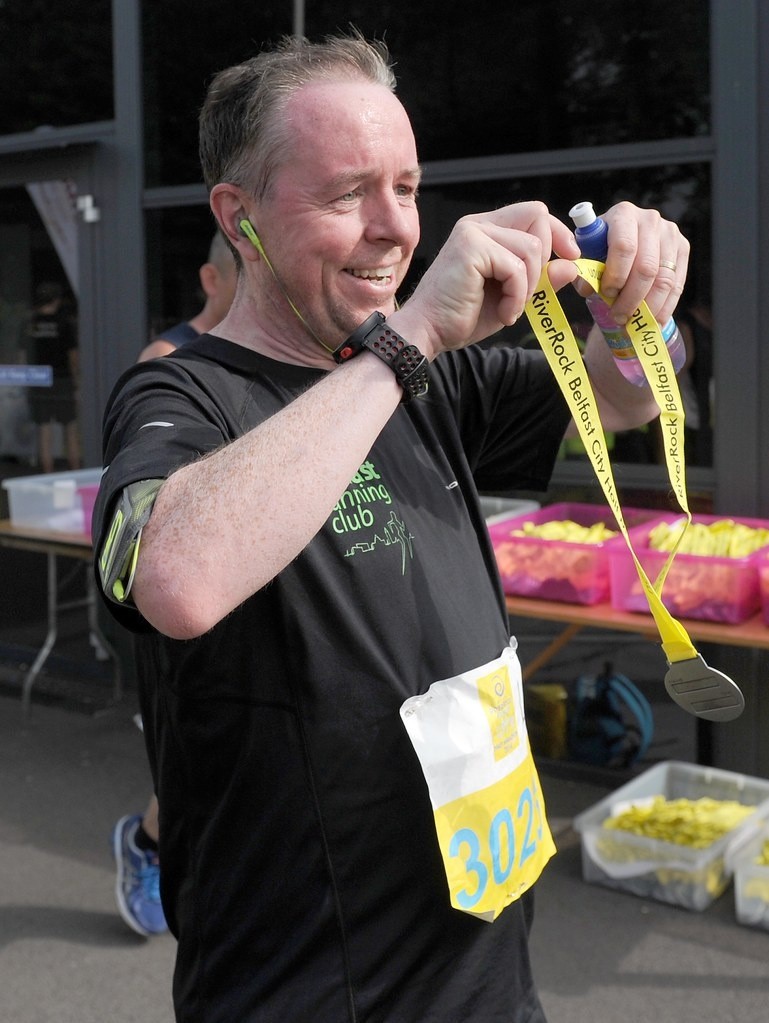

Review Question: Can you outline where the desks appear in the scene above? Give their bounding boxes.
[0,521,769,774]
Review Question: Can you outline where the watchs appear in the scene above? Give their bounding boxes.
[331,311,429,404]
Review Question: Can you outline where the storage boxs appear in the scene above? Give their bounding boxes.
[0,466,769,628]
[572,759,769,928]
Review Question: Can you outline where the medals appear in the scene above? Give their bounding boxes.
[662,654,745,722]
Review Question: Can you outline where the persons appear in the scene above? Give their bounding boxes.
[92,24,690,1023]
[16,282,82,474]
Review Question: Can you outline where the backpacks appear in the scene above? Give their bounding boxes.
[572,654,652,770]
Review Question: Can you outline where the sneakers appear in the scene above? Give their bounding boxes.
[111,815,168,938]
